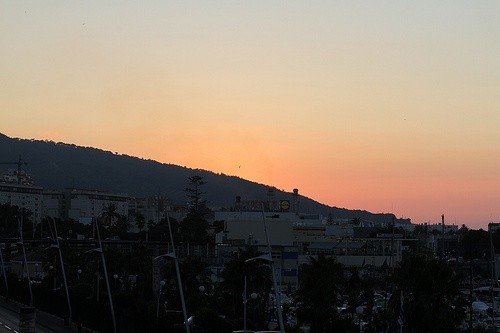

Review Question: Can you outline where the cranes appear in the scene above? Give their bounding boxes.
[0,155,28,187]
